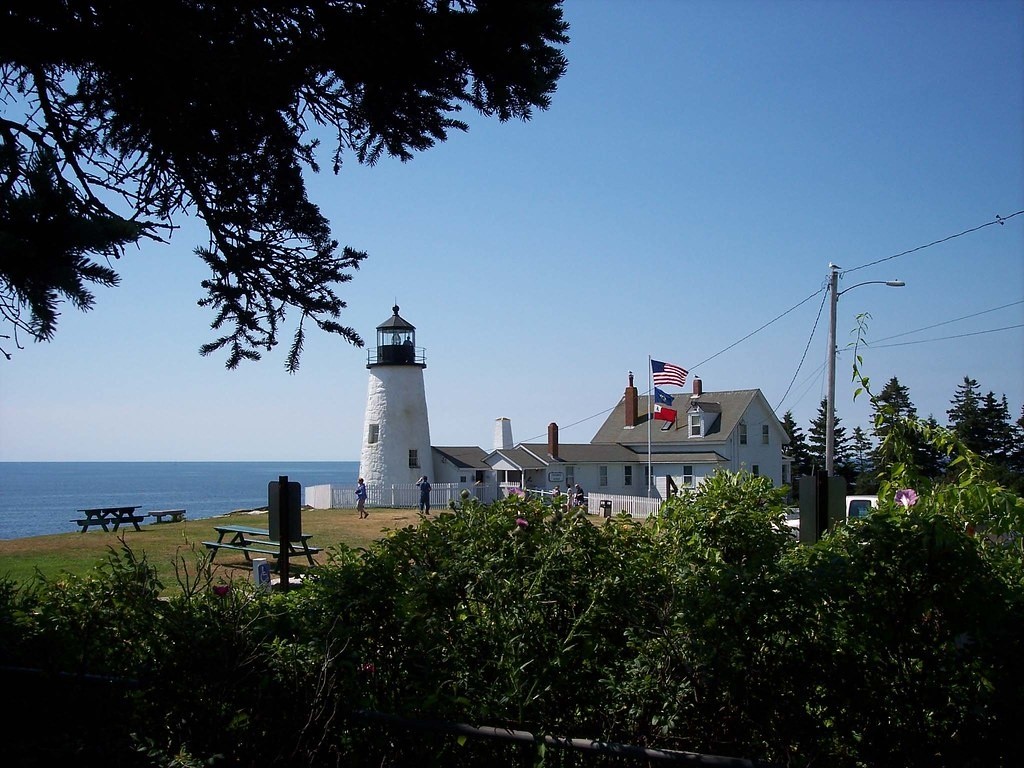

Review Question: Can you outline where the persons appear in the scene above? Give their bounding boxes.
[415,476,432,515]
[552,485,560,497]
[355,478,369,519]
[566,484,584,513]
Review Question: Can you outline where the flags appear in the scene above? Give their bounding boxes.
[653,404,677,423]
[655,387,674,406]
[651,359,689,388]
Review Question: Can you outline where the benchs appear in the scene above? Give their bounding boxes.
[244,538,324,555]
[148,509,186,524]
[69,518,111,526]
[110,514,150,524]
[201,541,280,559]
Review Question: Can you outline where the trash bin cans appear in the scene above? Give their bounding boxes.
[598,500,612,517]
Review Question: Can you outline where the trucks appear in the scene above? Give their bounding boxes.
[785,494,883,541]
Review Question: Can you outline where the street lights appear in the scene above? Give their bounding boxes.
[821,278,907,475]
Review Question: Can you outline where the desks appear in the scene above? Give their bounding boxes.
[77,506,142,533]
[208,525,315,573]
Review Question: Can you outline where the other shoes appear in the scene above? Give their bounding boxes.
[359,516,362,518]
[425,511,430,515]
[364,512,369,519]
